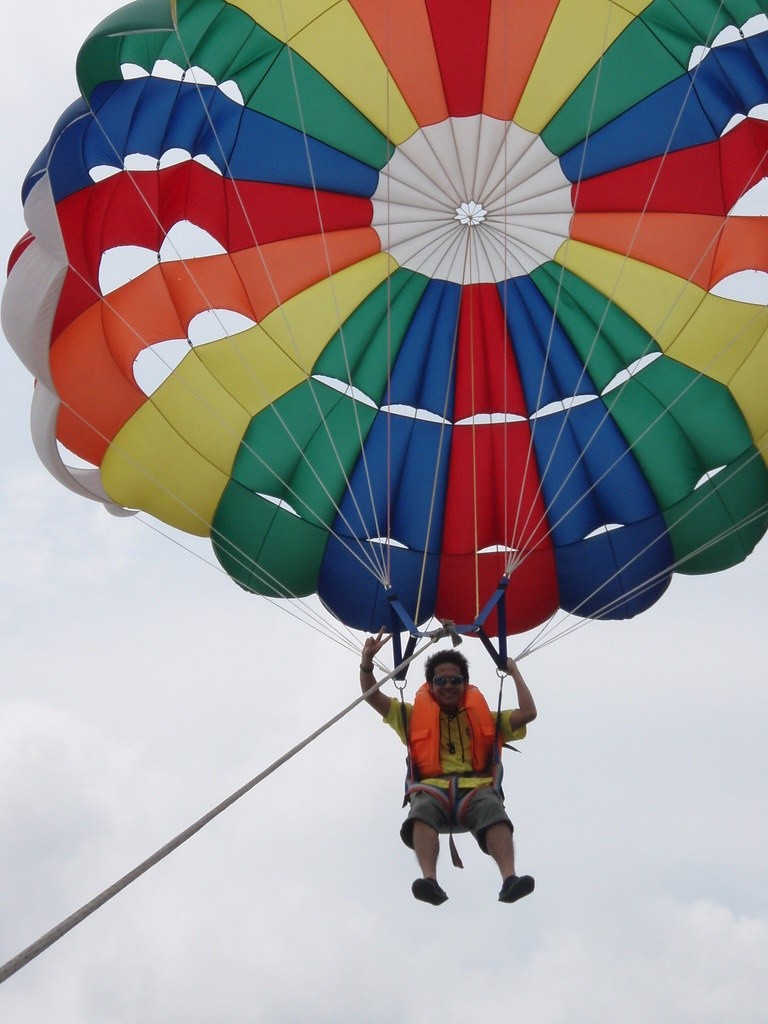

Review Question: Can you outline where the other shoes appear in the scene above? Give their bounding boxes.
[498,875,535,904]
[412,876,449,907]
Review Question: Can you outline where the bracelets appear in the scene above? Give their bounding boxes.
[360,663,374,672]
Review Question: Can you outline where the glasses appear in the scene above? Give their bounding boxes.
[432,675,465,686]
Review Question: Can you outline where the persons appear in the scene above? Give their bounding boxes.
[360,626,537,906]
